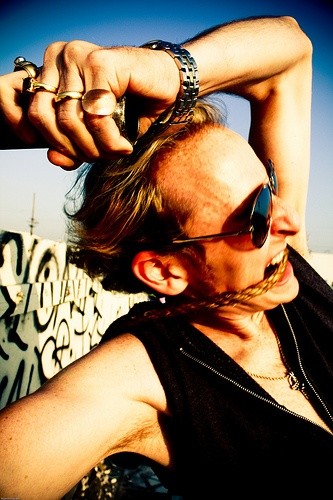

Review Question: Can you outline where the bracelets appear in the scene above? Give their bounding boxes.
[0,15,333,500]
[139,39,200,136]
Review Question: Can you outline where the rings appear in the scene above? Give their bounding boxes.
[26,78,58,94]
[13,56,39,77]
[82,89,116,116]
[54,91,83,102]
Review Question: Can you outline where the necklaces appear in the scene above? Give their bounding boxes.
[244,326,310,400]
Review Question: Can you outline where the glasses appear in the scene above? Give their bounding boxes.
[172,159,278,249]
